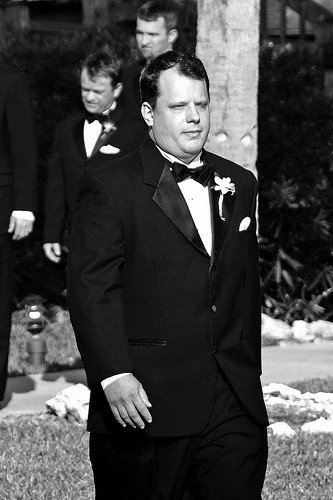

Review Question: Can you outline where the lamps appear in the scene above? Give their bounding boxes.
[29,306,41,319]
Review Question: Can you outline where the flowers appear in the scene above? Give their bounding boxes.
[210,171,236,223]
[101,121,117,140]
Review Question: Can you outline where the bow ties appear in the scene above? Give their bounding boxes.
[85,112,108,125]
[170,160,212,186]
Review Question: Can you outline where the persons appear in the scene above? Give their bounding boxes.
[43,50,154,297]
[68,49,268,500]
[114,0,179,147]
[0,60,39,405]
